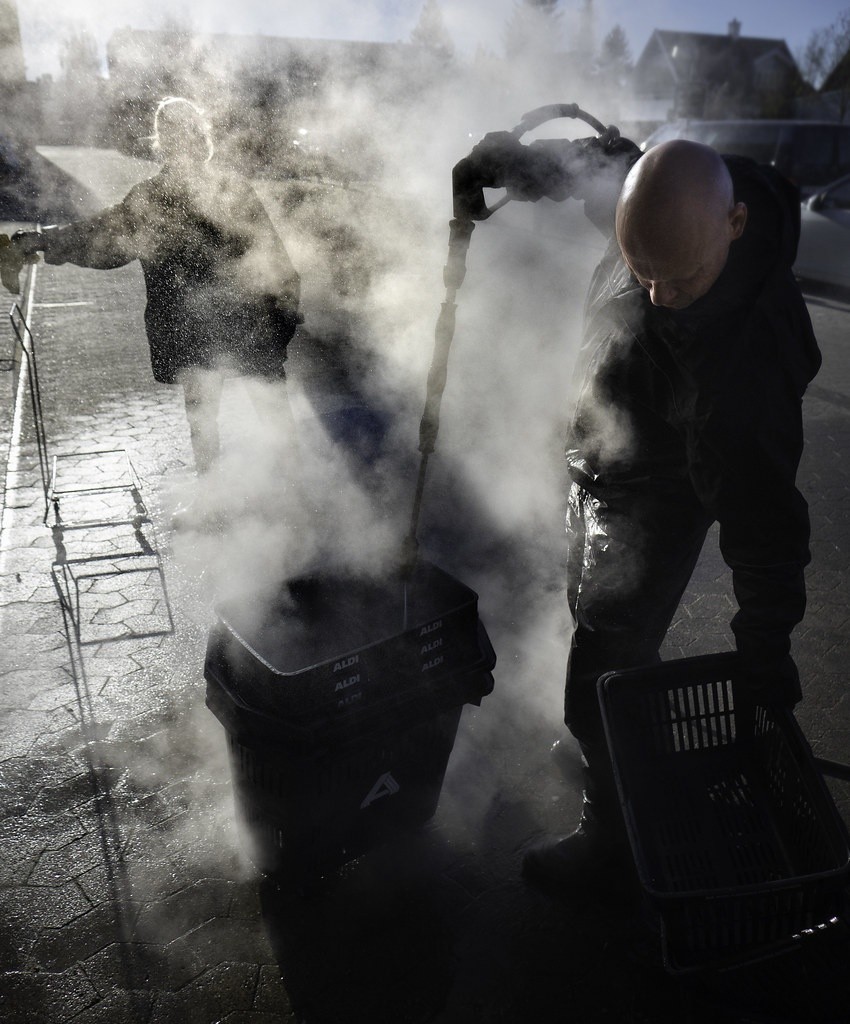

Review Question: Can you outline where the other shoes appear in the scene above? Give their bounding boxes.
[171,508,207,529]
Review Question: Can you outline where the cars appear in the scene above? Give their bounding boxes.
[788,174,850,289]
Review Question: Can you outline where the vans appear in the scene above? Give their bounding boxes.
[635,115,850,186]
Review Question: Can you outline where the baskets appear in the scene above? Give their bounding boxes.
[596,651,850,977]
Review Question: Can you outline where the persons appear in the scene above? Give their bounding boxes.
[12,98,302,525]
[472,127,821,890]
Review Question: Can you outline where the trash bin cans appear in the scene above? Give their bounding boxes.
[202,554,498,874]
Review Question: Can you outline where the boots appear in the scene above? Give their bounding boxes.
[522,765,631,896]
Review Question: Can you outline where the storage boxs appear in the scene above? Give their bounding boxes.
[597,652,850,987]
